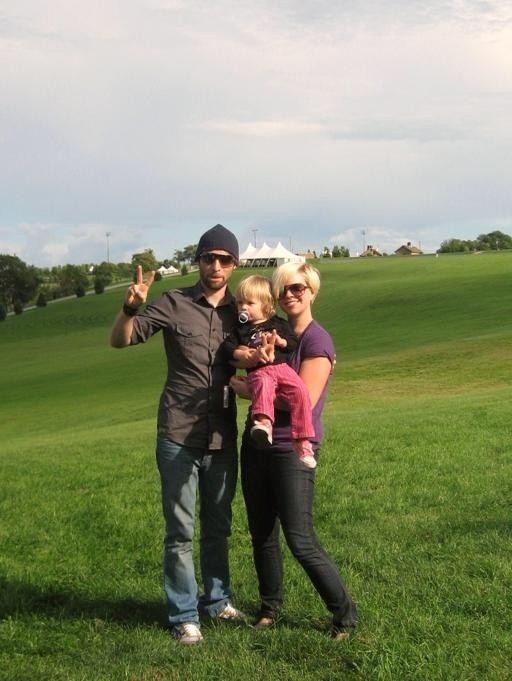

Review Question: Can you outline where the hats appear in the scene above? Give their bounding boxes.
[195,224,239,261]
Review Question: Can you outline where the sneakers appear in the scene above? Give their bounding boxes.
[292,439,317,468]
[173,623,204,643]
[332,632,348,642]
[220,604,244,619]
[250,419,273,446]
[255,616,273,631]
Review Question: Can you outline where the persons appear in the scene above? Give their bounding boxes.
[239,261,355,643]
[108,223,336,644]
[222,271,317,469]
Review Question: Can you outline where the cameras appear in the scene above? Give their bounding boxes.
[222,385,229,409]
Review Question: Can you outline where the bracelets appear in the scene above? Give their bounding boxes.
[123,304,137,317]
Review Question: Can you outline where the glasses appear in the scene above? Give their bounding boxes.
[198,252,235,268]
[279,283,311,298]
